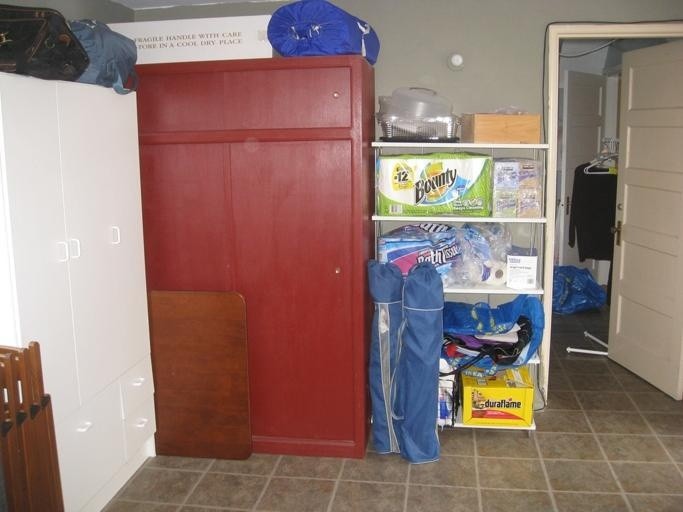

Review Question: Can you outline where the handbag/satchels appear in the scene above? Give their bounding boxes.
[71,13,140,97]
[0,3,88,84]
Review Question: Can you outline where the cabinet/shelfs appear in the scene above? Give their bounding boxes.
[370,140,555,424]
[128,55,378,458]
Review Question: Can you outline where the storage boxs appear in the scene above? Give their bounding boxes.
[458,364,534,428]
[505,245,538,290]
[438,372,454,428]
[461,111,542,145]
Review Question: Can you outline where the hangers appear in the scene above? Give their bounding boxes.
[590,140,616,169]
[583,138,618,175]
